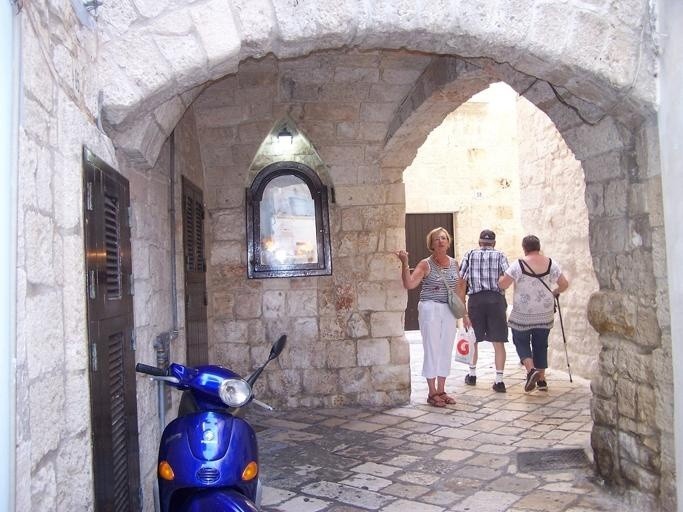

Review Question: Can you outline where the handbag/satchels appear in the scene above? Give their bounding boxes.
[447,289,466,319]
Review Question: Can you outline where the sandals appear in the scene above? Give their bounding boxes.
[438,391,457,405]
[426,392,446,407]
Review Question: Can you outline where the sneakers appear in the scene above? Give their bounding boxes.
[524,368,540,392]
[536,380,549,391]
[464,374,477,386]
[491,381,506,393]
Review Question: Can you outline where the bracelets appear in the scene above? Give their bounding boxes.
[464,315,468,317]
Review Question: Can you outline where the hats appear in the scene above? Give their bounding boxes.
[480,229,496,240]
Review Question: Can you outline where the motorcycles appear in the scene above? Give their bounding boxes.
[133,333,288,512]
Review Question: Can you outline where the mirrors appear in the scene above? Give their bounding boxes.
[244,161,332,279]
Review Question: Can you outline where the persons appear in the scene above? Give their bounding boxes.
[493,232,568,394]
[457,227,506,395]
[394,226,472,408]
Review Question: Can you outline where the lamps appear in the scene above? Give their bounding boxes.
[276,127,292,139]
